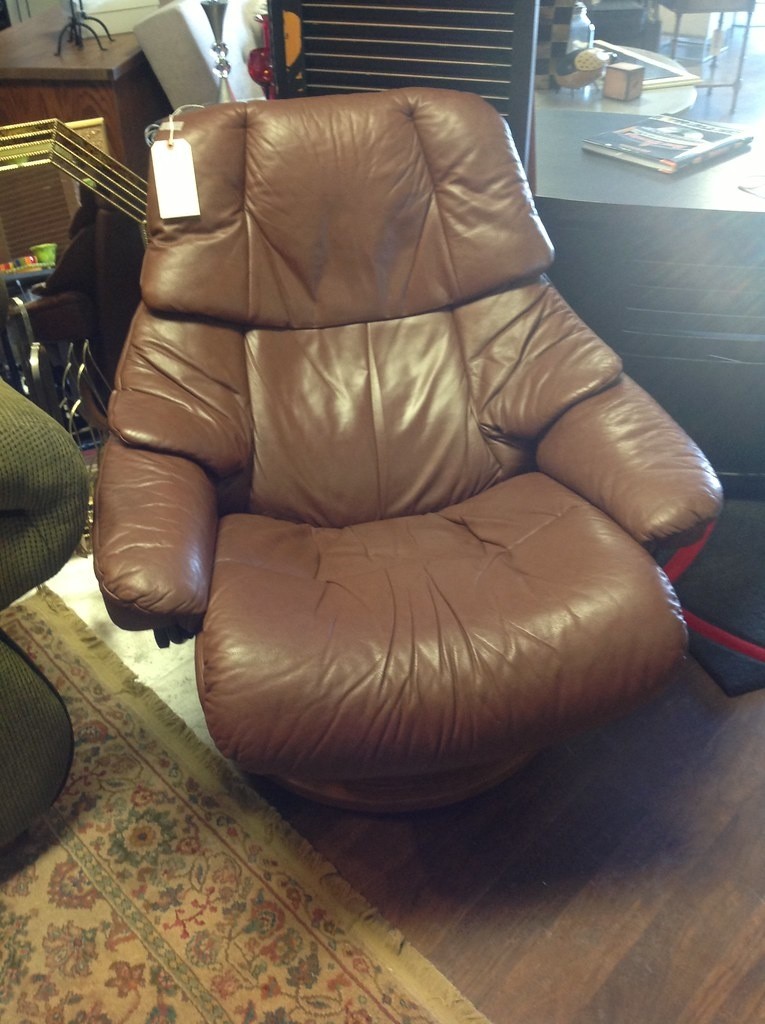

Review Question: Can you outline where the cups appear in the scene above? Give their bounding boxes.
[14,256,37,272]
[0,262,14,271]
[30,242,56,263]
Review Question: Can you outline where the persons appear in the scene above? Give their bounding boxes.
[221,0,269,100]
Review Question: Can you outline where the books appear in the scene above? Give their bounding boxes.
[581,112,754,174]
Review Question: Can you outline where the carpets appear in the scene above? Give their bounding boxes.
[0,576,498,1024]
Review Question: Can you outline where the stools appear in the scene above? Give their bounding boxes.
[656,0,758,112]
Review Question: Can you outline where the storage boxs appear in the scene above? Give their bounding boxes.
[602,59,646,101]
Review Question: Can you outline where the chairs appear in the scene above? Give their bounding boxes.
[88,89,733,827]
[0,365,94,858]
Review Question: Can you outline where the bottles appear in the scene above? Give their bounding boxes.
[566,4,595,53]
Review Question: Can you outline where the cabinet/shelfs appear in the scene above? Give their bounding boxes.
[528,103,765,504]
